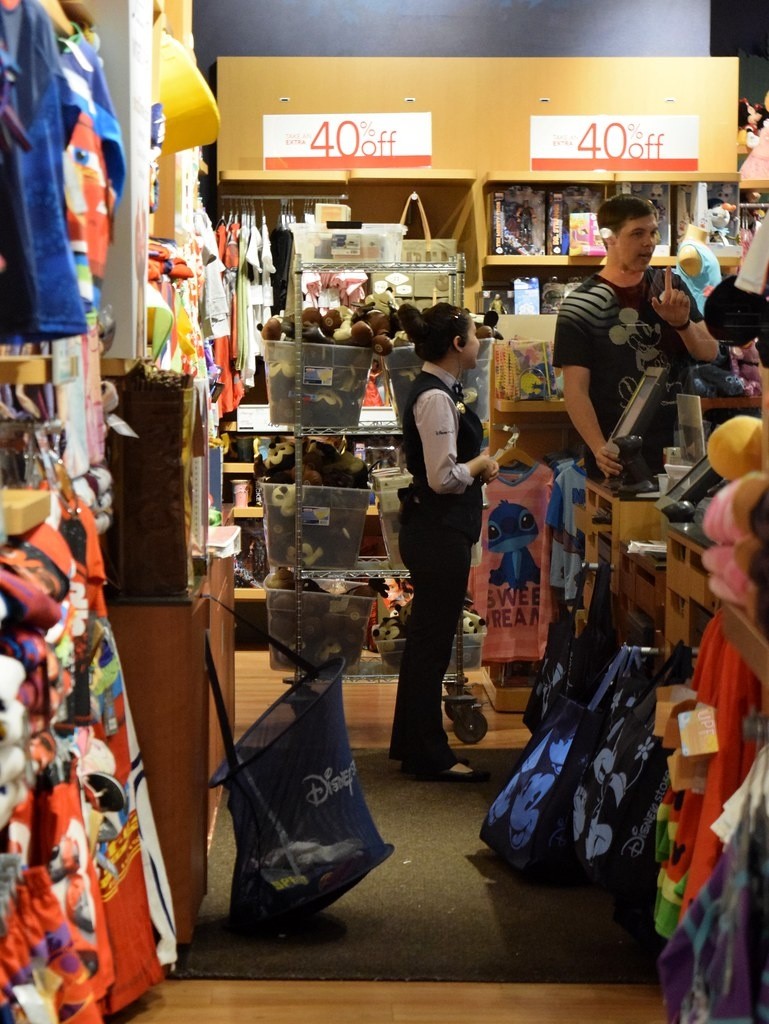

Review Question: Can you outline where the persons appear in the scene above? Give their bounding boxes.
[552,193,722,479]
[676,223,720,319]
[362,359,384,407]
[516,199,537,245]
[389,300,501,780]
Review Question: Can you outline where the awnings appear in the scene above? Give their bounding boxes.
[738,91,769,180]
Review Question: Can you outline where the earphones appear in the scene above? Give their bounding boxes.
[458,340,465,347]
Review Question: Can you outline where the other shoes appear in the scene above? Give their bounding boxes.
[407,763,490,783]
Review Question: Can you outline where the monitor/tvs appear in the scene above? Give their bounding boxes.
[664,454,724,505]
[603,366,669,458]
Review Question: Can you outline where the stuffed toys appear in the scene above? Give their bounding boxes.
[708,197,739,245]
[689,343,762,398]
[252,435,488,673]
[262,287,493,426]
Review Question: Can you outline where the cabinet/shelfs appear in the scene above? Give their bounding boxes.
[215,173,485,602]
[100,556,234,947]
[485,171,769,451]
[576,479,769,824]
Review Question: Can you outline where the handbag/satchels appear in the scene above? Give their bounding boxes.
[478,646,640,878]
[523,563,613,734]
[371,191,457,311]
[572,642,693,876]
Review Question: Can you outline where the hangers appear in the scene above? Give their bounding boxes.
[479,426,537,471]
[217,194,344,228]
[1,415,77,514]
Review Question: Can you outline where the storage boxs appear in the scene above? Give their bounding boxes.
[262,339,372,429]
[378,338,494,428]
[257,476,375,571]
[373,469,415,569]
[371,625,486,674]
[262,573,376,677]
[286,222,408,265]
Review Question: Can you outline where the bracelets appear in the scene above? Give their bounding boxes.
[672,318,690,330]
